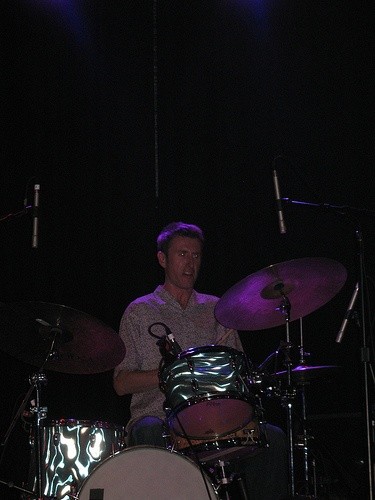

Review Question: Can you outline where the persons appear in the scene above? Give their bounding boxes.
[113,221,292,500]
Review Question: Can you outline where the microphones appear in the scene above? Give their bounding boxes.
[334,284,359,343]
[273,168,286,235]
[30,183,42,249]
[167,333,182,355]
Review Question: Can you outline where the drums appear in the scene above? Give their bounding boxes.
[161,344,258,440]
[164,419,266,466]
[18,419,127,500]
[75,444,223,500]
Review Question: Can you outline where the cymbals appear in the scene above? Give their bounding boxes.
[266,364,343,380]
[214,254,348,331]
[0,301,126,375]
